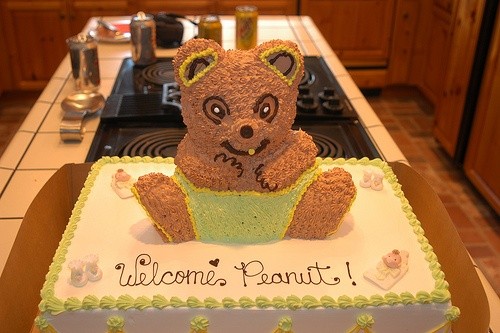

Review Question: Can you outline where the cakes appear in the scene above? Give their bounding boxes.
[33,38,460,333]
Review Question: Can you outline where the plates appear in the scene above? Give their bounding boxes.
[89,20,131,43]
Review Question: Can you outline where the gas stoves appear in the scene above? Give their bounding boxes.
[82,54,383,164]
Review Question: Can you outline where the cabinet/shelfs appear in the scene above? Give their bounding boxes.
[0,1,394,95]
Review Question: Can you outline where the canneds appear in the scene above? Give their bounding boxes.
[68,34,100,92]
[130,11,159,65]
[235,6,258,50]
[197,15,222,48]
